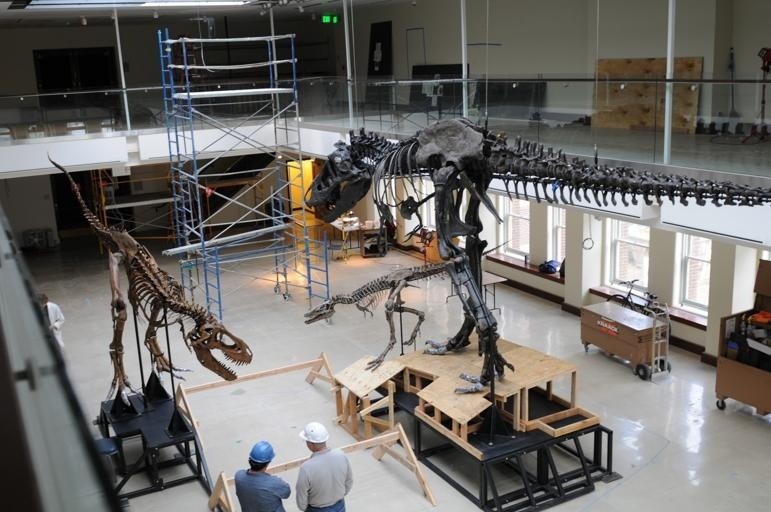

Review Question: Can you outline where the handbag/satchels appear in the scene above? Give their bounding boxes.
[539,260,560,273]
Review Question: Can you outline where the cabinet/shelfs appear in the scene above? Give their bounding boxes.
[361,225,387,257]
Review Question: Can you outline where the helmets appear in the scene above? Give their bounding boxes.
[250,441,274,463]
[300,423,329,443]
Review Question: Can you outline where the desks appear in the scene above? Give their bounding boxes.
[93,394,224,512]
[332,219,387,257]
[445,272,508,315]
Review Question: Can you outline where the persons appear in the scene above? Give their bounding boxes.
[295,422,353,512]
[38,294,65,352]
[235,441,291,512]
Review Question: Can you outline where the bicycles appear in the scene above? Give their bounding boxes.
[605,279,670,322]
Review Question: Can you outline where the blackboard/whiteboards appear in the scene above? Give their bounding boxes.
[33,46,121,108]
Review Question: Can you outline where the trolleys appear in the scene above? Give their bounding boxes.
[711,304,771,423]
